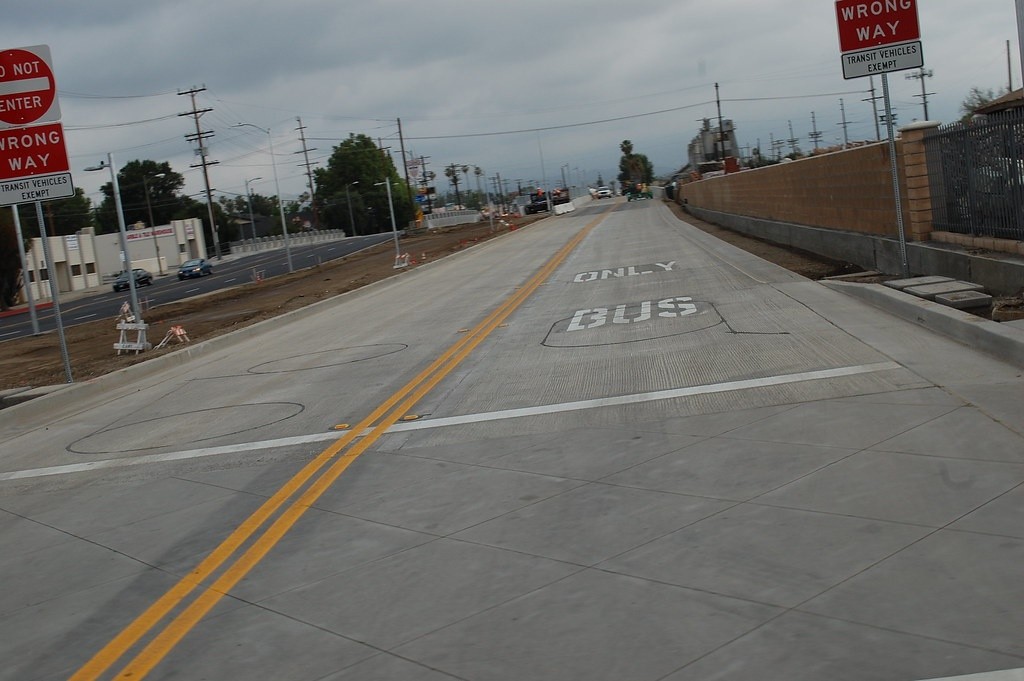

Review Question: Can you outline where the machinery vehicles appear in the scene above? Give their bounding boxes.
[524,190,553,215]
[551,187,570,204]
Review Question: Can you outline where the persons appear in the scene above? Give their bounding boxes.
[537,187,542,202]
[588,185,597,199]
[552,188,561,197]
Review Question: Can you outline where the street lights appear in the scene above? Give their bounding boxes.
[244,177,264,242]
[83,151,152,353]
[374,176,401,266]
[345,181,359,236]
[142,173,167,276]
[230,122,293,274]
[561,165,568,189]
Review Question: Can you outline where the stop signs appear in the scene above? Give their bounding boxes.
[0,44,62,130]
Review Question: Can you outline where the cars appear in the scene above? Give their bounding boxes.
[177,257,214,281]
[112,268,153,292]
[597,186,612,199]
